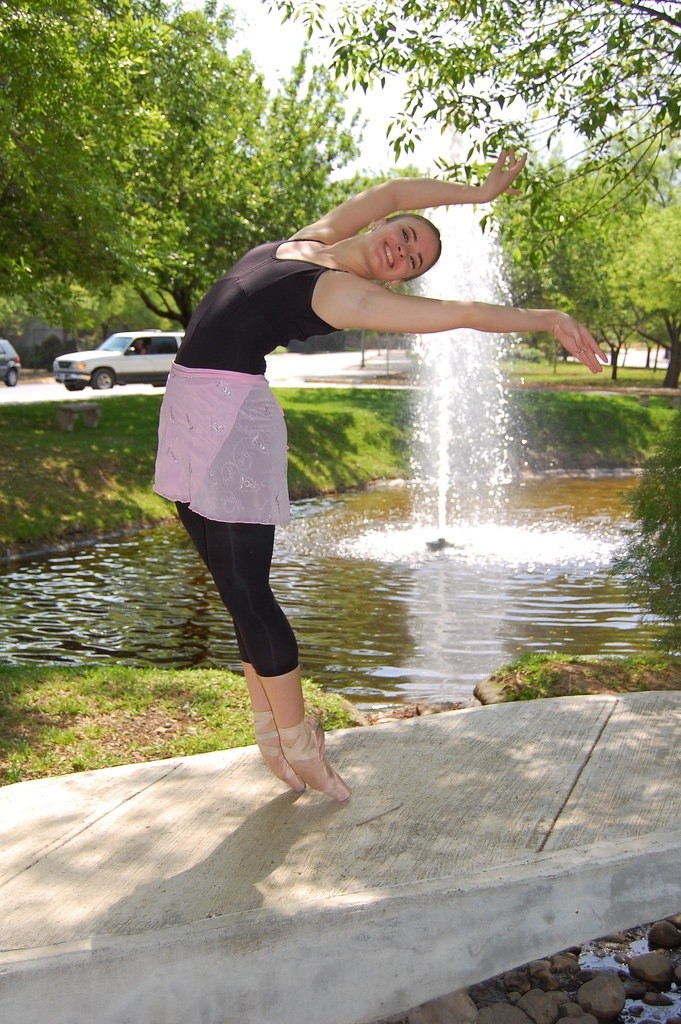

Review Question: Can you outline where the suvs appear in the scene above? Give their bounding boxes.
[52,329,186,392]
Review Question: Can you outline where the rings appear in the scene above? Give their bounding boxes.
[577,350,584,353]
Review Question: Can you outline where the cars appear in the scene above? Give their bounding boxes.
[0,339,22,386]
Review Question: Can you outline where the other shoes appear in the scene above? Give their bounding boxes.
[253,710,352,801]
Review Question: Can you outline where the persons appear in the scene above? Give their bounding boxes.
[148,146,608,801]
[134,339,146,354]
